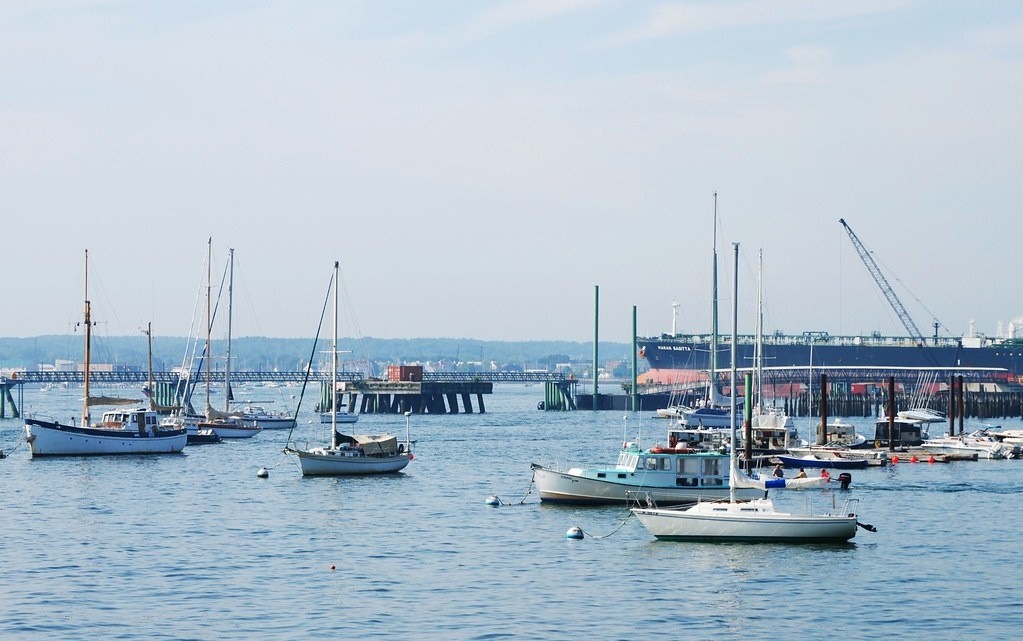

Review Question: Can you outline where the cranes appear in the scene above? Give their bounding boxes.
[838,217,948,380]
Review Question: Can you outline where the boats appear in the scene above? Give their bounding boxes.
[319,407,360,423]
[530,449,770,504]
[655,394,1023,469]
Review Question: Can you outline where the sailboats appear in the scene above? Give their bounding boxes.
[630,242,860,541]
[283,261,417,476]
[26,237,298,456]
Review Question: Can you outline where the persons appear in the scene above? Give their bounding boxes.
[773,464,783,478]
[835,426,843,438]
[821,469,830,482]
[690,397,711,408]
[796,466,806,478]
